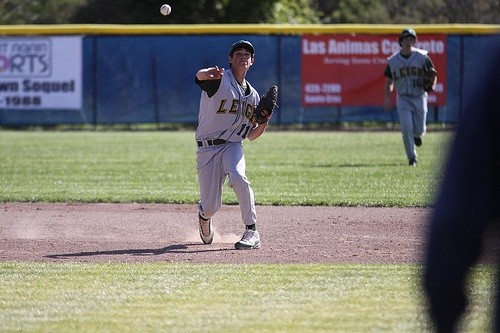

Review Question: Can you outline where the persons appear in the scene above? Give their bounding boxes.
[381,28,437,168]
[194,40,277,250]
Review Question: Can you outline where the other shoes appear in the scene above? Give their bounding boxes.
[409,159,416,168]
[415,136,422,146]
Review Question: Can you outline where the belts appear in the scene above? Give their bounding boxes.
[198,139,226,147]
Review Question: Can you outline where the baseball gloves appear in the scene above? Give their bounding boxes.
[254,85,278,125]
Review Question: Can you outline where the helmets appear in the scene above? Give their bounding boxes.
[229,39,255,55]
[399,29,416,47]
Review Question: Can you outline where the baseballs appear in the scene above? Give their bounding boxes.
[160,4,172,16]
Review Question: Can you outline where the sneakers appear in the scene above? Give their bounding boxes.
[199,211,214,244]
[235,229,260,249]
